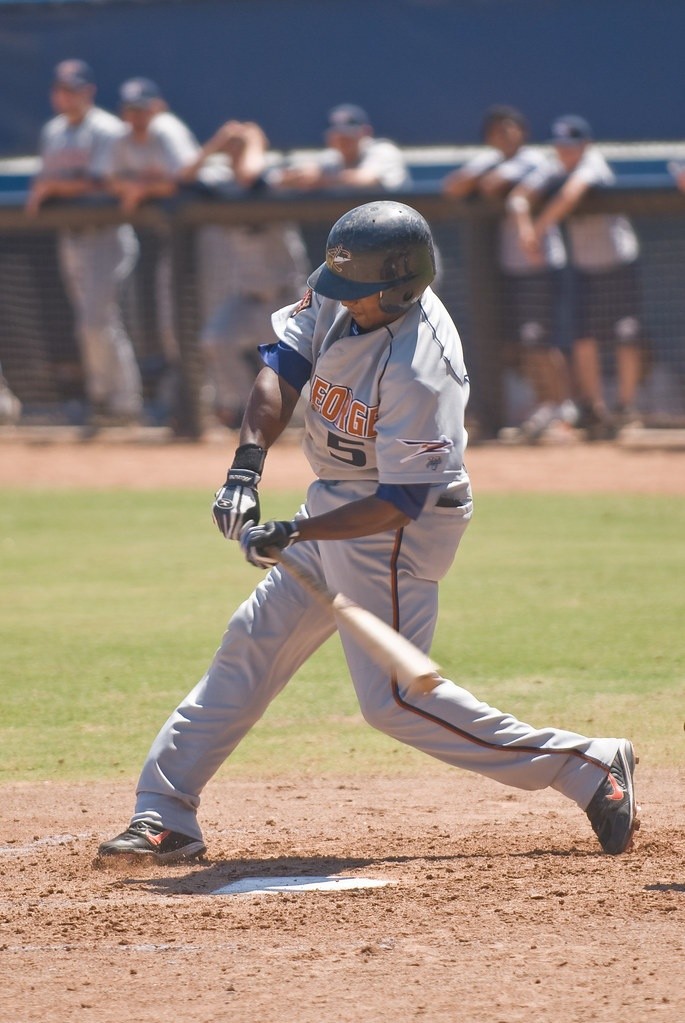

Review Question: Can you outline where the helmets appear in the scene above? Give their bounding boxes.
[307,200,437,314]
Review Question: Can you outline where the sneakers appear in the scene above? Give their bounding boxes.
[584,738,641,855]
[98,821,207,866]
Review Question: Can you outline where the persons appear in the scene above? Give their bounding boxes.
[98,201,639,862]
[440,106,651,452]
[24,57,141,429]
[105,76,442,448]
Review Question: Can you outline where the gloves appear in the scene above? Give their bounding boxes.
[238,519,300,569]
[211,469,261,541]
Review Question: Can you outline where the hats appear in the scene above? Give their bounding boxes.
[52,60,90,90]
[322,104,371,139]
[549,115,591,147]
[118,78,161,109]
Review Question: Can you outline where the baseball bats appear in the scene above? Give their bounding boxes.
[263,539,442,699]
